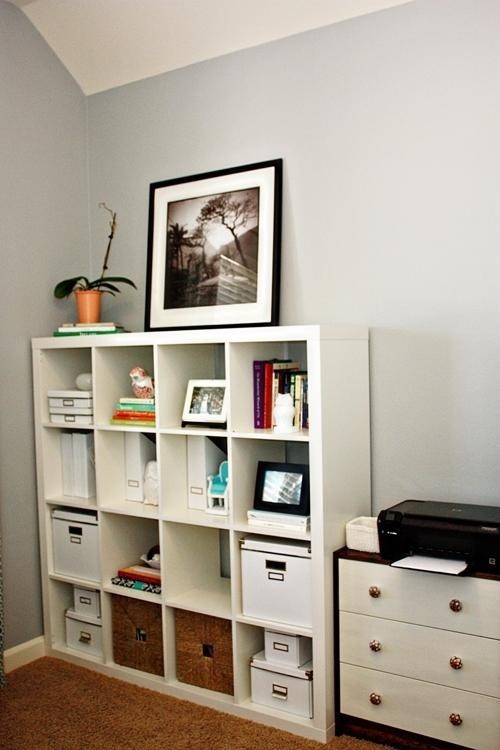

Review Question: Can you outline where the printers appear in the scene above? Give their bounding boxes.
[376,499,500,582]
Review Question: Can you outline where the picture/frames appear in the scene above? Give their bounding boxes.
[253,460,309,515]
[181,379,226,427]
[145,157,283,332]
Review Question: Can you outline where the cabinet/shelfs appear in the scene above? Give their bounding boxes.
[29,324,372,747]
[338,557,500,750]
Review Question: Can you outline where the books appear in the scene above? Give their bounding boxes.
[248,519,310,533]
[246,510,310,526]
[110,397,156,428]
[255,358,308,429]
[53,330,132,336]
[58,327,124,331]
[62,323,121,326]
[111,561,161,594]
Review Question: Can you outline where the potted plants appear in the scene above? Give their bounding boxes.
[53,275,138,325]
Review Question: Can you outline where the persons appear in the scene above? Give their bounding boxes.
[198,393,211,414]
